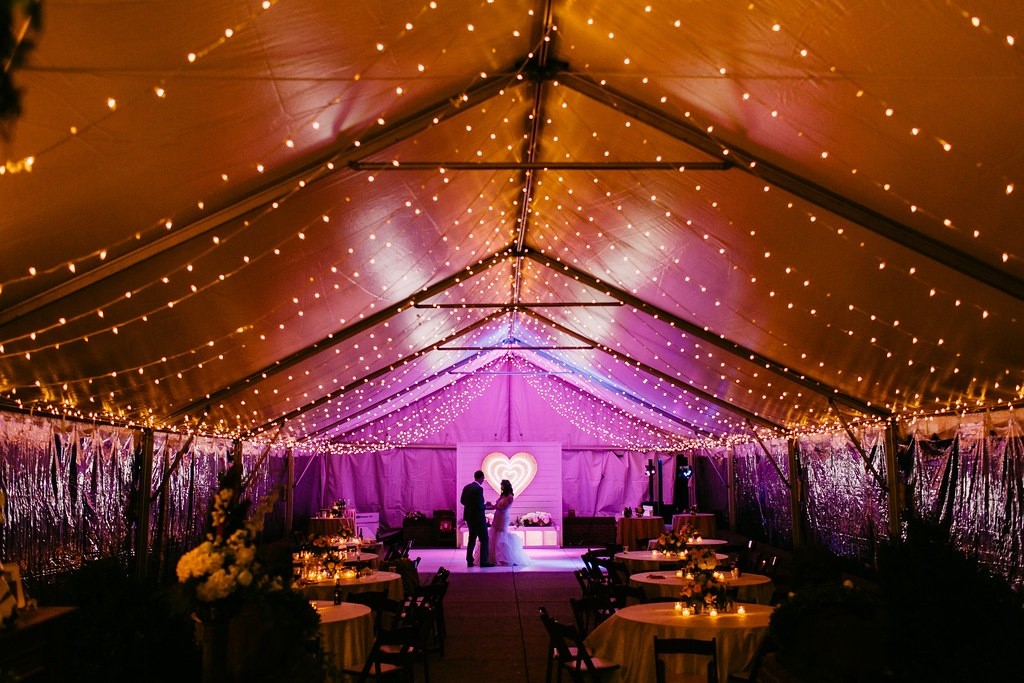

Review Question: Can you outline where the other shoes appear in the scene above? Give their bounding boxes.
[467,563,494,568]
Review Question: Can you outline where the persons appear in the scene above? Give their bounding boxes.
[473,479,533,567]
[460,469,496,568]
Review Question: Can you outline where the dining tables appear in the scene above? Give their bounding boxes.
[1,504,782,683]
